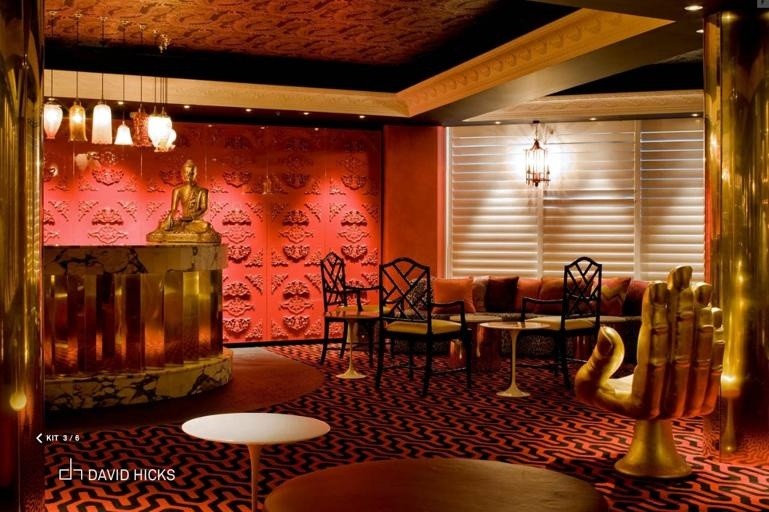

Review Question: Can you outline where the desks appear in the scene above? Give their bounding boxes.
[181,411,332,511]
[323,310,380,381]
[261,449,615,512]
[479,322,550,398]
[450,311,504,372]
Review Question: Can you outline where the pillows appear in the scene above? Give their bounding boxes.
[408,273,650,317]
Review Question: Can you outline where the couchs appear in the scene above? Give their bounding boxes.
[390,275,654,356]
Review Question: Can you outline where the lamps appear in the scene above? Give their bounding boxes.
[43,9,180,154]
[523,119,551,189]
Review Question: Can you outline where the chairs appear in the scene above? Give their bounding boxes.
[374,256,473,395]
[319,251,396,368]
[510,257,603,387]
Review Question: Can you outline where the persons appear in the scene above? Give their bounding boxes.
[145,160,222,243]
